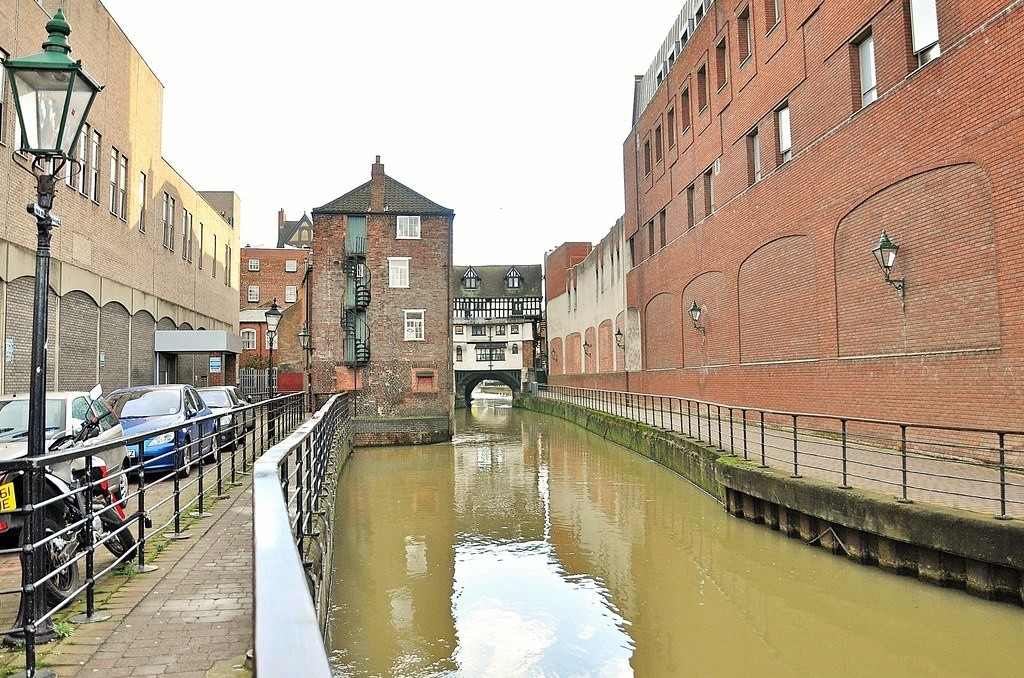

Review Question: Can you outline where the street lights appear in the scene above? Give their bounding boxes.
[265,298,283,440]
[0,6,107,647]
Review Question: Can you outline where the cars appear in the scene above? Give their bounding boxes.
[103,384,218,478]
[0,391,131,534]
[183,389,247,451]
[201,386,256,432]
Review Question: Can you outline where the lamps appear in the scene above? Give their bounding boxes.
[551,349,557,361]
[687,298,705,335]
[873,229,905,299]
[583,339,591,358]
[297,328,315,355]
[615,327,625,350]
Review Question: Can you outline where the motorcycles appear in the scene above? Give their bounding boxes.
[18,383,137,607]
